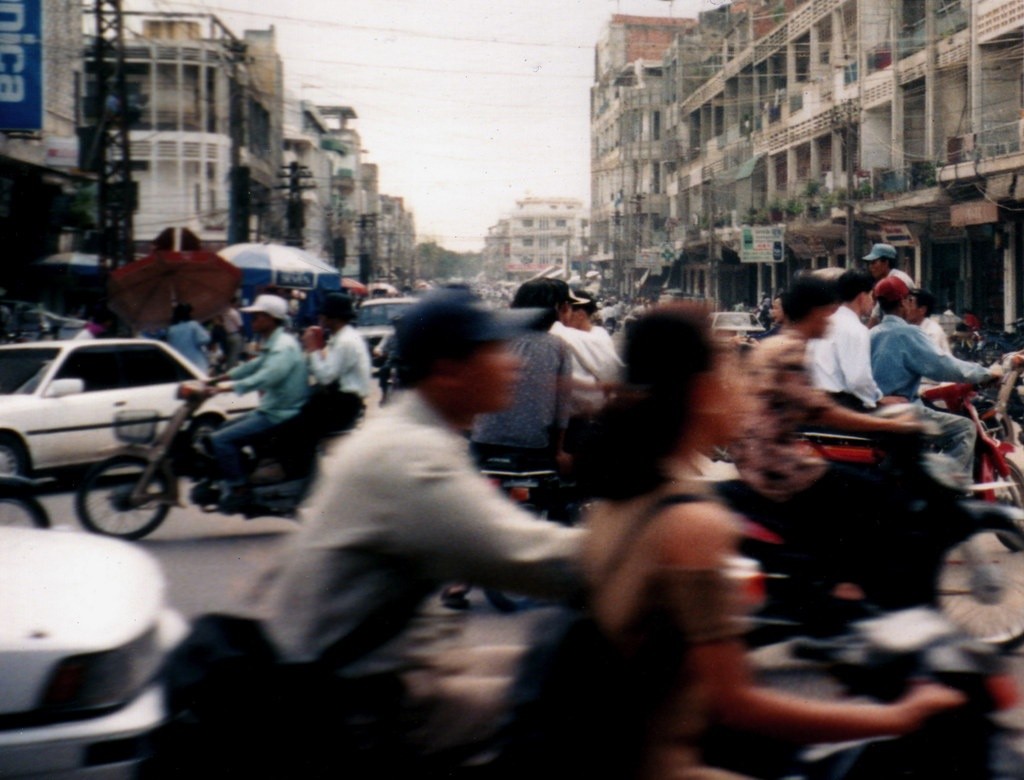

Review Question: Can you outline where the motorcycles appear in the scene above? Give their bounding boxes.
[0,474,58,528]
[370,318,1024,780]
[73,369,312,542]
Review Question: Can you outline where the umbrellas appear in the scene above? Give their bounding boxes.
[43,250,99,276]
[105,250,239,333]
[219,242,341,291]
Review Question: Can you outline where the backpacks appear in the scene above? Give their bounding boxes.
[495,492,709,780]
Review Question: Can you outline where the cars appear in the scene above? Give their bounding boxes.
[1,338,211,498]
[353,299,421,375]
[706,312,766,343]
[0,527,194,779]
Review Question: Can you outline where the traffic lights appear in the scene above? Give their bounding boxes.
[359,253,370,284]
[233,165,252,217]
[287,199,306,229]
[334,236,346,268]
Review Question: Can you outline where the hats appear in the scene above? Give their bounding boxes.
[547,276,587,303]
[240,295,290,321]
[874,276,920,303]
[863,242,899,263]
[395,285,546,378]
[320,294,358,322]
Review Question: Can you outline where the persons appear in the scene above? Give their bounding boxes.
[551,299,963,780]
[167,301,212,375]
[375,313,401,407]
[805,268,909,413]
[207,302,246,371]
[940,301,980,342]
[906,288,953,408]
[732,273,927,628]
[547,285,633,412]
[468,277,574,447]
[730,292,785,349]
[293,292,372,474]
[862,243,915,328]
[759,290,771,311]
[868,275,1003,474]
[180,293,309,507]
[258,287,591,755]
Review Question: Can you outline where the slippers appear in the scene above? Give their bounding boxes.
[442,588,467,609]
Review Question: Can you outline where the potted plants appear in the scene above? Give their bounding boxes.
[766,198,781,222]
